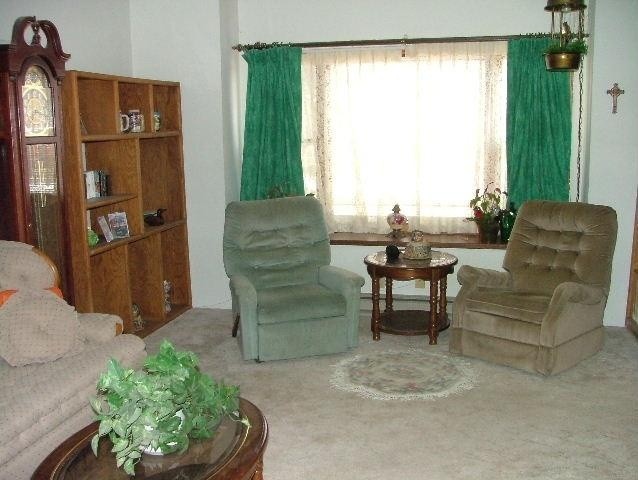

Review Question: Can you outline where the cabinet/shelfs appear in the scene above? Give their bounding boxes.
[64,70,193,342]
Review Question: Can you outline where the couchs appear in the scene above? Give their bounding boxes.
[0,240,146,480]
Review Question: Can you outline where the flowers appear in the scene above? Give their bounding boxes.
[478,224,501,242]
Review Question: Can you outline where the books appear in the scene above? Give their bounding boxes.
[81,142,87,172]
[81,170,111,200]
[108,211,130,240]
[95,215,115,244]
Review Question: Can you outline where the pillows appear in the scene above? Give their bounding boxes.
[1,289,80,364]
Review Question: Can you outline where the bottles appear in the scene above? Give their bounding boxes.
[129,109,141,133]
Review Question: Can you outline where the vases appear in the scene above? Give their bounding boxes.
[465,182,507,225]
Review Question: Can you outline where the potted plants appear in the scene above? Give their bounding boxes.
[544,37,589,73]
[93,345,244,476]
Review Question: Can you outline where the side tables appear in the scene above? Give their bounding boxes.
[365,248,457,345]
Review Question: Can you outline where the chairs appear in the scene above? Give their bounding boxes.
[450,200,619,377]
[221,195,367,362]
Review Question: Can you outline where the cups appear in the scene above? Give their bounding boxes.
[120,110,130,132]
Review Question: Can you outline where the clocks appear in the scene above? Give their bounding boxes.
[0,16,71,285]
